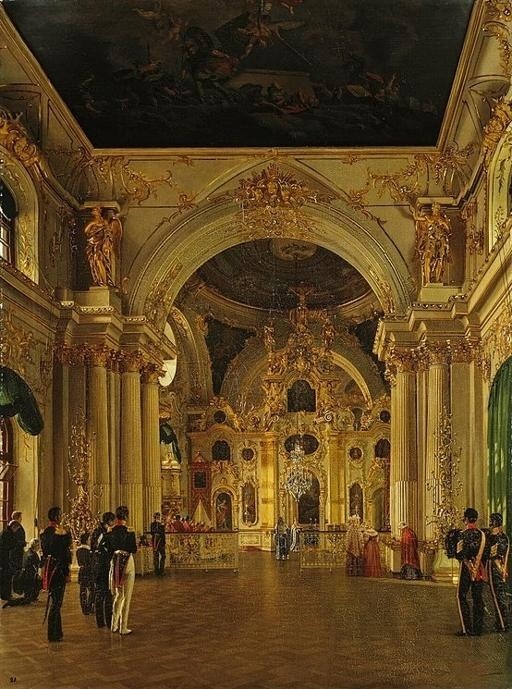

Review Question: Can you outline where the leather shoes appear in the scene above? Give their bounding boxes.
[84,608,132,635]
[455,622,512,636]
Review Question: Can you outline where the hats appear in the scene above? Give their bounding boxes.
[366,529,377,537]
[397,521,407,529]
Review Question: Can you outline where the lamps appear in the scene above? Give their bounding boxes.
[282,442,312,504]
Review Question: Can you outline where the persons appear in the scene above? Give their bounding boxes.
[151,512,166,575]
[321,320,339,355]
[217,500,229,530]
[455,507,486,636]
[487,512,509,632]
[171,515,214,532]
[263,321,275,353]
[346,524,363,576]
[398,522,418,579]
[351,495,361,516]
[415,204,454,287]
[365,530,382,578]
[0,507,138,641]
[276,518,290,560]
[85,208,114,286]
